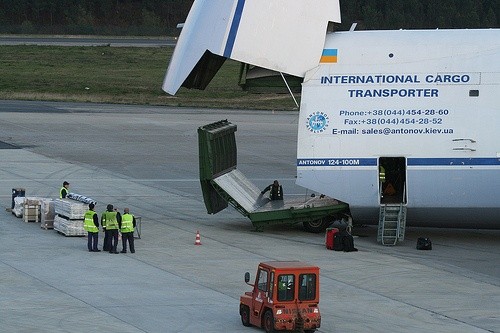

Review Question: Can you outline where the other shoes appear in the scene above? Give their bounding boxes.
[121,251,126,253]
[93,249,101,252]
[115,252,119,254]
[89,250,93,252]
[131,250,135,253]
[109,251,113,253]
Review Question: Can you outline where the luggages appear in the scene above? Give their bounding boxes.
[343,234,354,251]
[332,232,344,251]
[326,228,340,250]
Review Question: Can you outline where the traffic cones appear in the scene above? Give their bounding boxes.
[193,229,202,245]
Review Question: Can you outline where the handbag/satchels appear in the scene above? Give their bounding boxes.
[416,238,432,250]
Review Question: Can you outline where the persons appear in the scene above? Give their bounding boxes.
[101,204,121,253]
[83,203,101,252]
[59,181,69,199]
[271,276,283,291]
[119,208,136,253]
[379,162,385,188]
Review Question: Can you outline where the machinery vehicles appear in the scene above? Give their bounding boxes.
[239,261,321,333]
